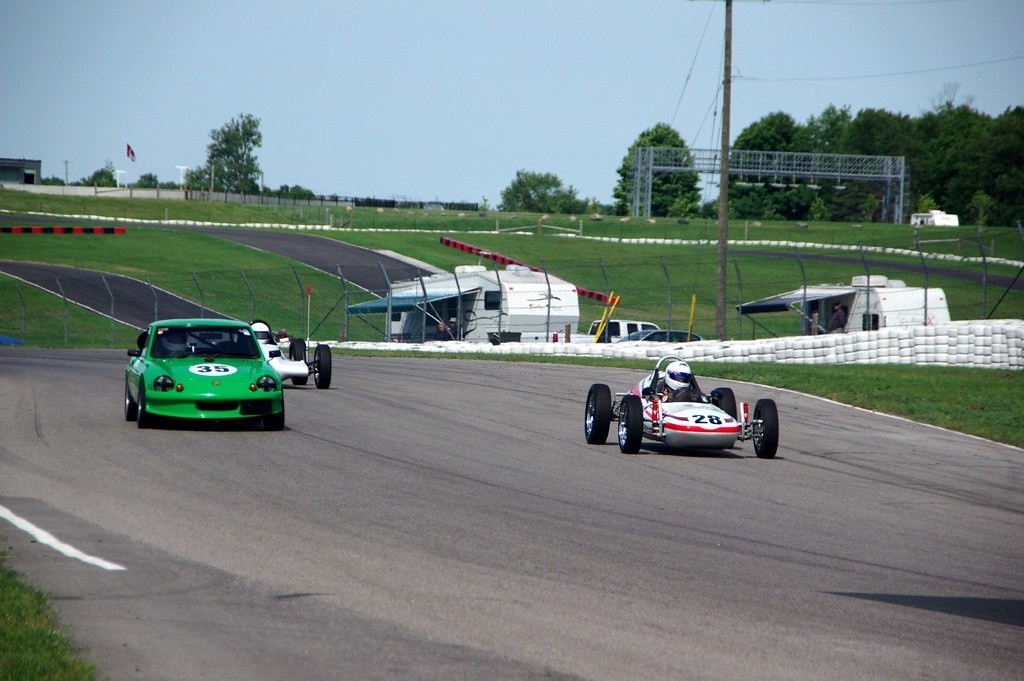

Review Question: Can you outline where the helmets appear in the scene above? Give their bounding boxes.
[250,321,270,343]
[665,361,692,391]
[161,332,187,351]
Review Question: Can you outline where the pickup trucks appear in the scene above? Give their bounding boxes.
[520,319,660,346]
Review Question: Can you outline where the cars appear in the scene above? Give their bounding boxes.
[124,318,287,432]
[616,329,706,345]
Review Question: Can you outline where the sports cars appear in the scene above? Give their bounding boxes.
[245,318,332,391]
[582,356,784,458]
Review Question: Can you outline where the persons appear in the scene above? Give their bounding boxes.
[660,361,700,402]
[828,300,847,332]
[434,322,450,341]
[449,316,462,340]
[248,322,270,345]
[158,330,190,356]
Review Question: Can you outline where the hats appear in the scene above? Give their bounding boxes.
[834,300,841,305]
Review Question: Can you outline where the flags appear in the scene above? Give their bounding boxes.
[306,284,311,296]
[125,143,136,161]
